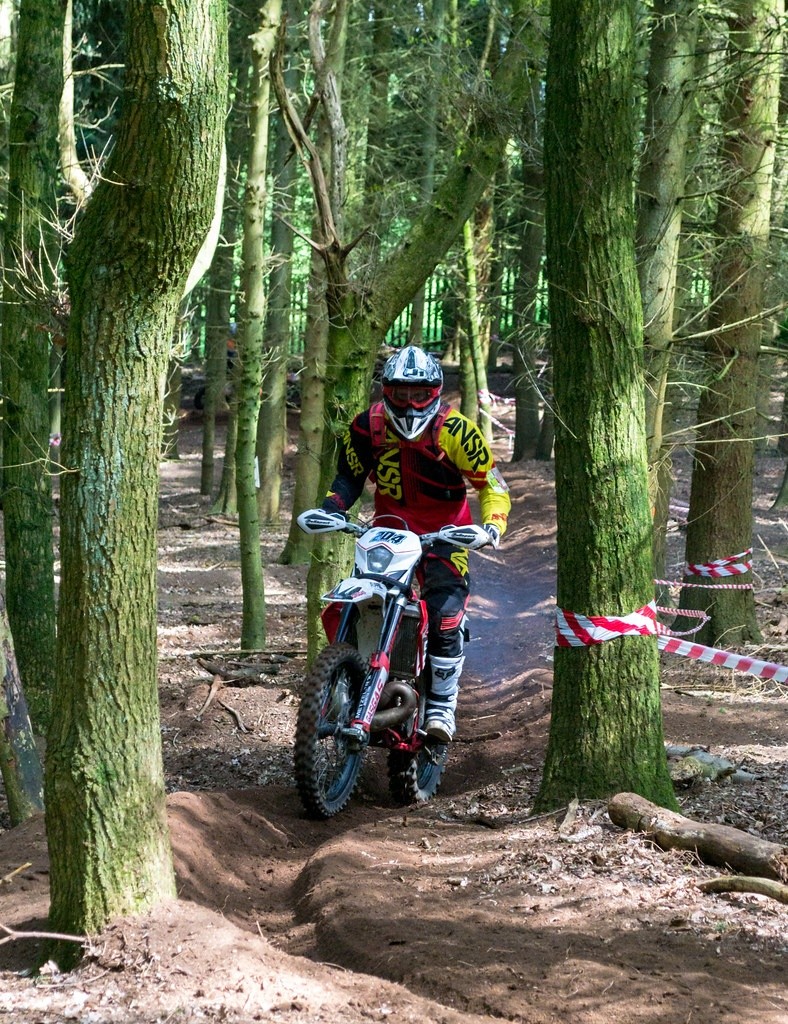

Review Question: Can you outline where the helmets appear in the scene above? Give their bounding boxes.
[381,346,444,439]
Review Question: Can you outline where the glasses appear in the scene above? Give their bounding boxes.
[382,384,441,407]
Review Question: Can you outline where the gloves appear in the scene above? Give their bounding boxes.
[480,524,500,544]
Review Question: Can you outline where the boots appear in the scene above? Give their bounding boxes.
[331,669,349,714]
[428,654,465,742]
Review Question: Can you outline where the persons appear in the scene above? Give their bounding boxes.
[227,317,240,371]
[322,346,511,743]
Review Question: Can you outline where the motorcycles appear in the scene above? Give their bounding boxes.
[293,507,494,821]
[190,342,302,412]
